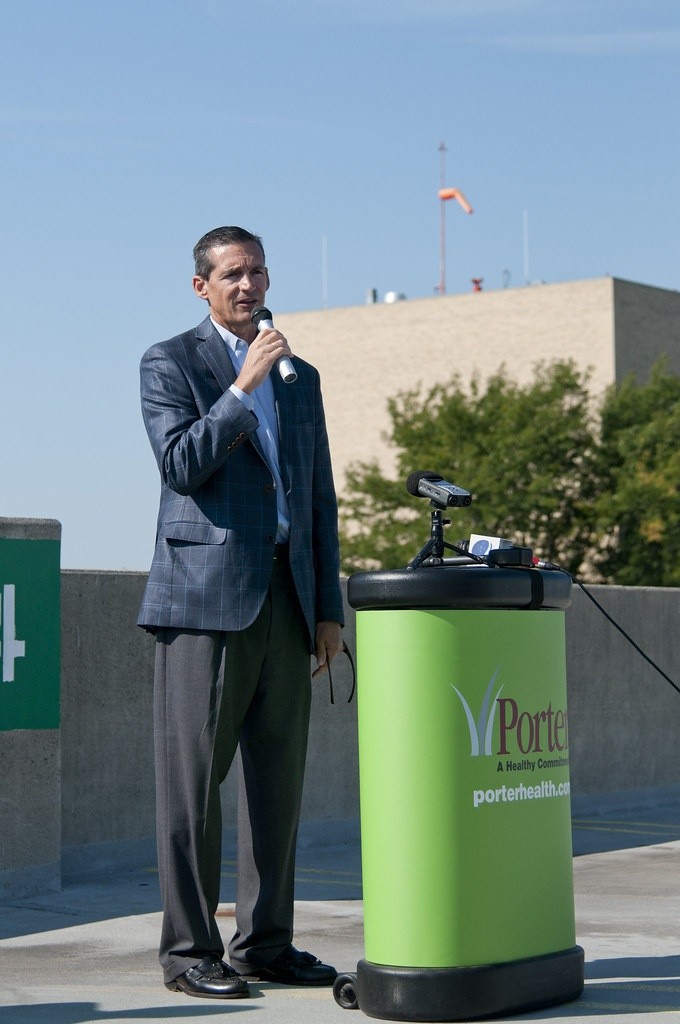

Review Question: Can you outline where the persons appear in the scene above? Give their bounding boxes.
[136,226,347,1001]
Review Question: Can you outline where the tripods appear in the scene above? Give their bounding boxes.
[406,510,483,572]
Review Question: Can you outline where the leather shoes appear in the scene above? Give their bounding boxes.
[164,959,250,997]
[237,944,337,985]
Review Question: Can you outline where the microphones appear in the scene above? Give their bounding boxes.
[406,471,472,510]
[532,556,557,571]
[250,305,298,383]
